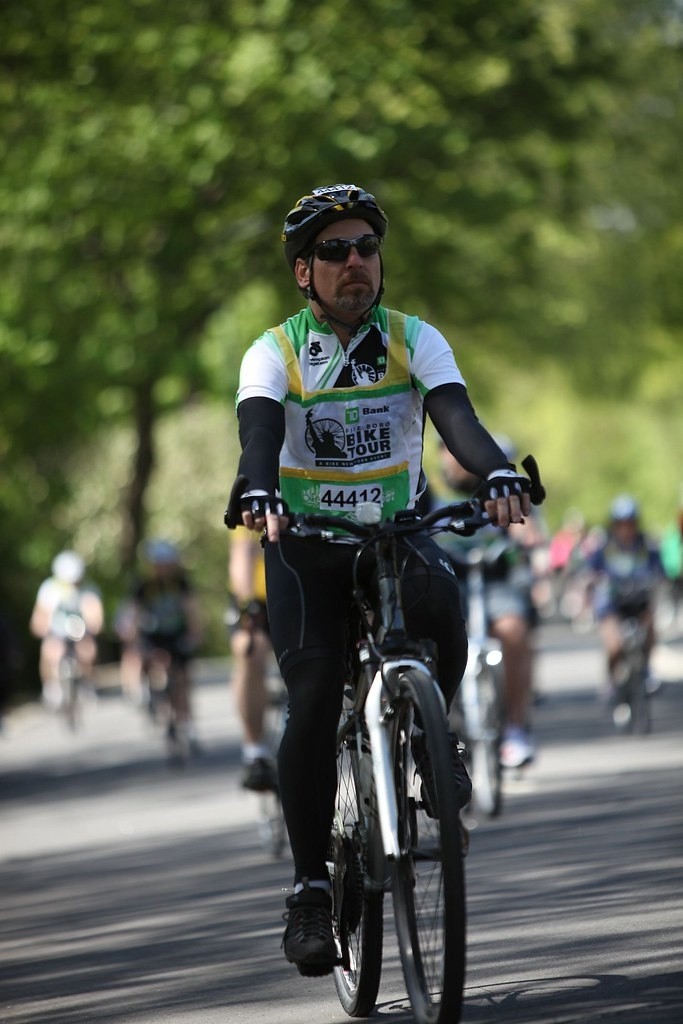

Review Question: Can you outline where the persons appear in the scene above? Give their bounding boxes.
[549,496,683,701]
[433,437,540,767]
[224,182,532,978]
[228,493,278,793]
[27,553,103,705]
[118,539,202,723]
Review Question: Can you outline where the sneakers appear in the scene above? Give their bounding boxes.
[409,731,472,820]
[280,876,334,964]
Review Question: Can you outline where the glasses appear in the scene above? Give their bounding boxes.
[310,233,384,262]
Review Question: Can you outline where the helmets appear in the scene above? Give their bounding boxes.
[610,493,638,524]
[51,549,86,585]
[281,183,388,276]
[143,541,178,567]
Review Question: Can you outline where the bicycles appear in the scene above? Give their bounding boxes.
[261,453,547,1024]
[52,578,657,858]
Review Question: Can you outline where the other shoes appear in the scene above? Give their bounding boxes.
[497,725,535,769]
[241,743,277,771]
